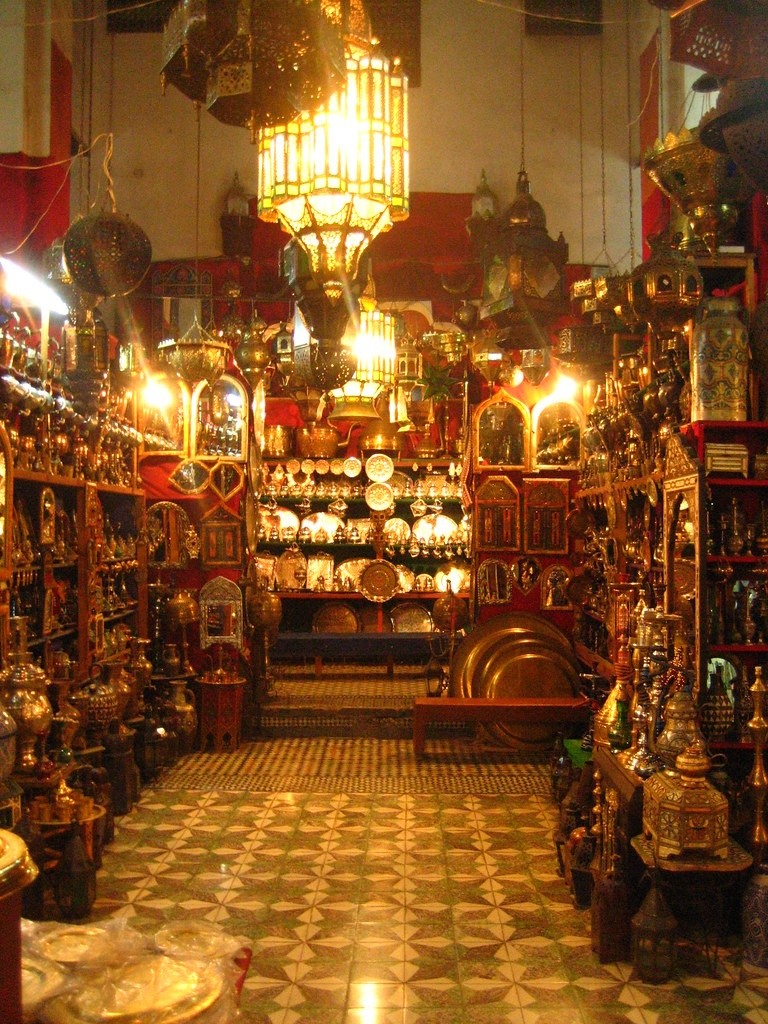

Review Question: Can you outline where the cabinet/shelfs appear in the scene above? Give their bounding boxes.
[0,241,768,956]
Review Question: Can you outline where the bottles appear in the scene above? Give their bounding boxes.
[53,636,196,778]
[700,674,734,739]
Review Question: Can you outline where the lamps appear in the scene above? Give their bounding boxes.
[257,0,412,303]
[479,14,571,348]
[324,277,403,421]
